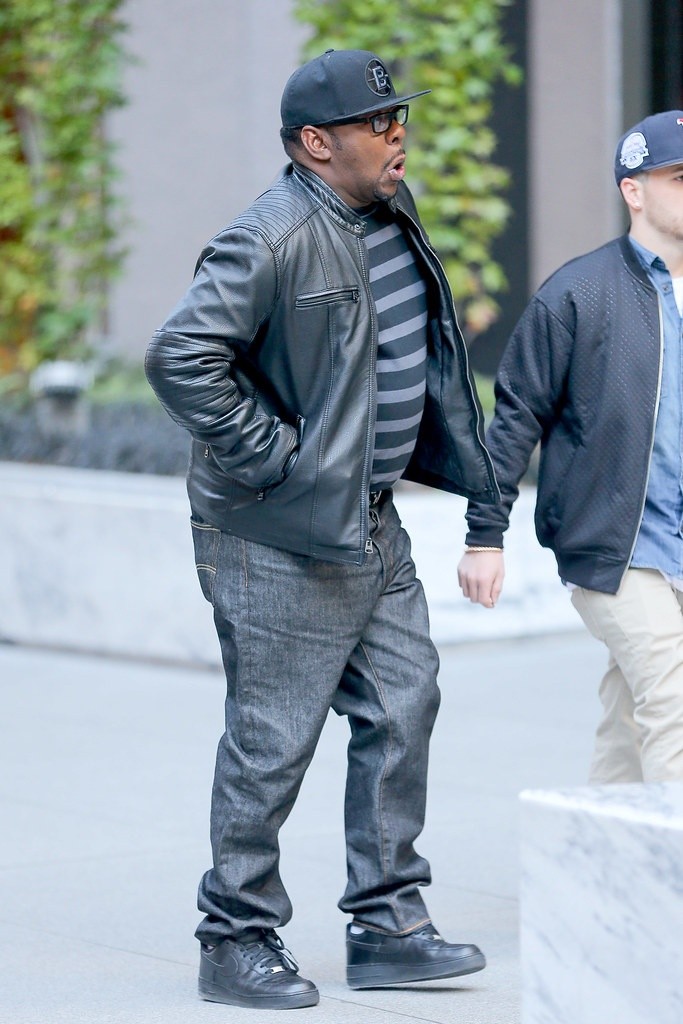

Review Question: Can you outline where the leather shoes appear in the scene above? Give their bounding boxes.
[345,922,486,990]
[197,940,320,1010]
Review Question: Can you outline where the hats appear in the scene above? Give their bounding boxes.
[280,48,433,129]
[615,109,682,185]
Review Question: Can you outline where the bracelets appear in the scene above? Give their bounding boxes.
[464,546,502,552]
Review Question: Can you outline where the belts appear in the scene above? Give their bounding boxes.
[368,489,391,507]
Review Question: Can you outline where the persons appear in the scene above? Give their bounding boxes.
[456,110,683,789]
[144,49,500,1010]
